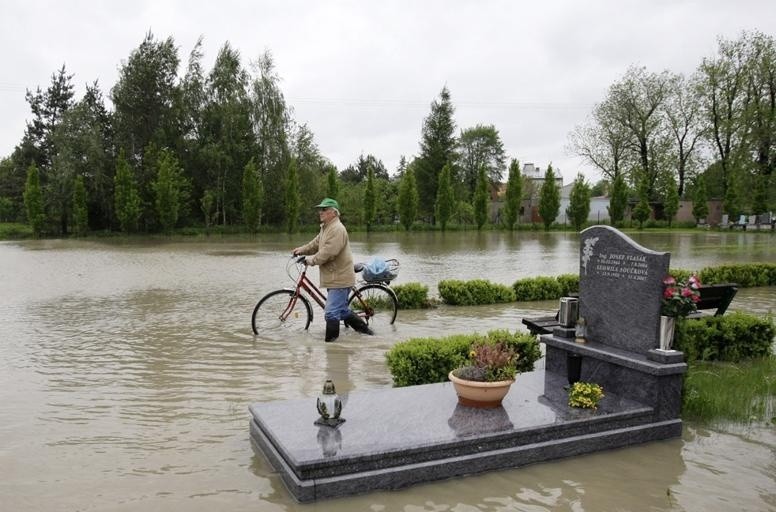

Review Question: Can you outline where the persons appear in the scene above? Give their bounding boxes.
[290,197,375,342]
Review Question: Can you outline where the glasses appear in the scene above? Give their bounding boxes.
[319,208,327,211]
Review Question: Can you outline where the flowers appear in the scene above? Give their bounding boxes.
[563,381,606,410]
[662,275,703,317]
[457,342,521,381]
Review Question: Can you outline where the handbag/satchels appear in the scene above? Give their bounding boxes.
[363,256,400,283]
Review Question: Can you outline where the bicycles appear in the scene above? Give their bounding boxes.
[251,250,400,335]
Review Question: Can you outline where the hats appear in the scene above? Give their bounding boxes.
[314,197,338,209]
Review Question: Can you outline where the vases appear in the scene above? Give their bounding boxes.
[448,368,514,407]
[660,315,676,349]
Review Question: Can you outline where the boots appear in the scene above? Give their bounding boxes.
[325,320,339,342]
[346,310,374,336]
